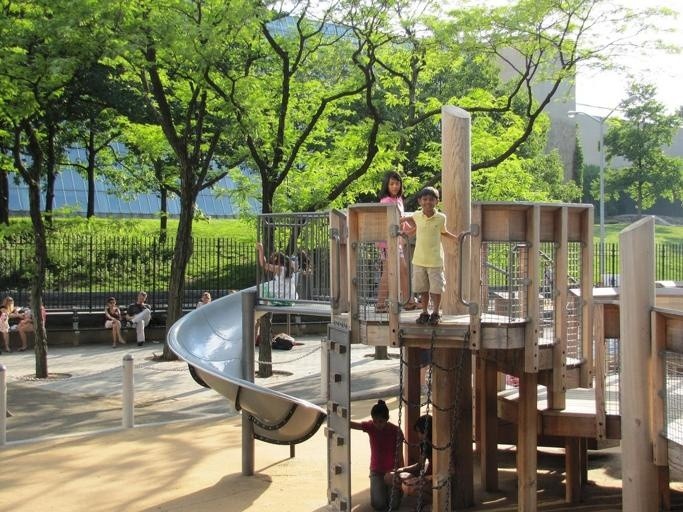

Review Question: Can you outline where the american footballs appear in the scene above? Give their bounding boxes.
[401,215,416,236]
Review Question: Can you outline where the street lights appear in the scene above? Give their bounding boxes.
[566,93,641,286]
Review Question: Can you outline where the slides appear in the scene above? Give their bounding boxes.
[165,278,327,445]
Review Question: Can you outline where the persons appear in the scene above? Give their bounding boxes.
[349,400,404,512]
[125,291,152,346]
[256,242,299,306]
[104,296,125,348]
[399,187,464,325]
[374,171,422,313]
[196,292,211,310]
[291,250,311,288]
[383,414,457,505]
[16,293,47,352]
[0,296,17,352]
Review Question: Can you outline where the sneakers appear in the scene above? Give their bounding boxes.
[416,312,430,325]
[428,312,440,327]
